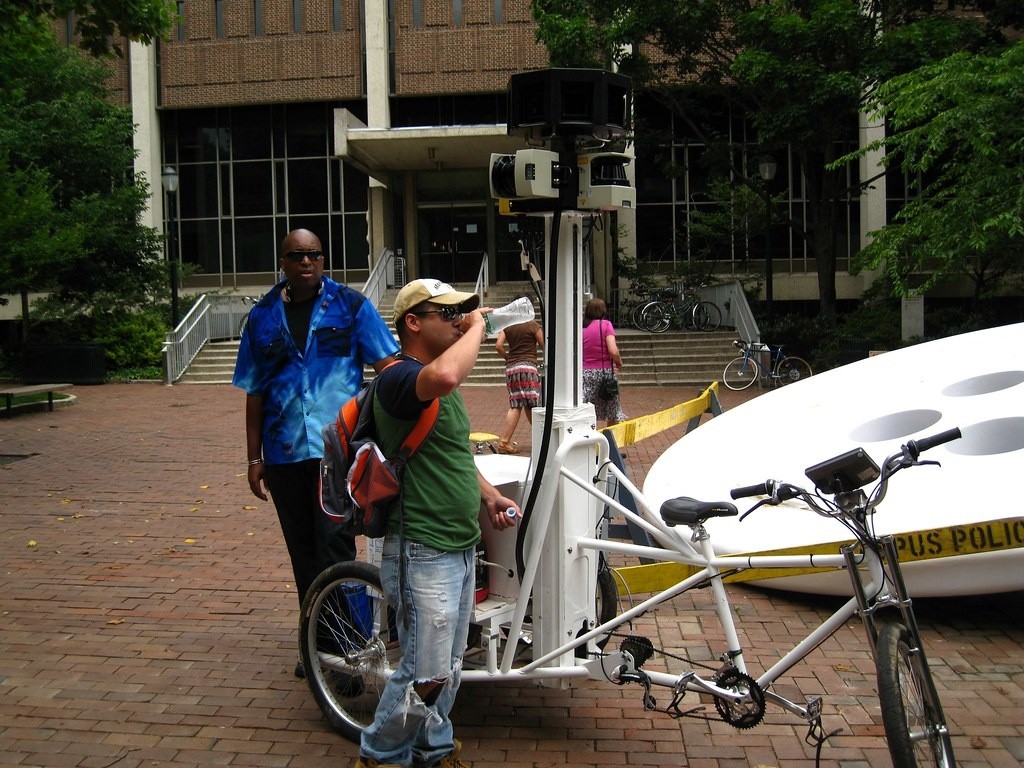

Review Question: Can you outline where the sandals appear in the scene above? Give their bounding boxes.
[498,440,521,454]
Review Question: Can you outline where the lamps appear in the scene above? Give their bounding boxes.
[428,147,435,158]
[437,161,442,173]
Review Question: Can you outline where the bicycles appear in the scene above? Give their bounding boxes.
[722,340,813,391]
[631,287,723,333]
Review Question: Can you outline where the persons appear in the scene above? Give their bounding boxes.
[233,228,400,700]
[495,293,545,454]
[355,278,524,768]
[583,298,628,463]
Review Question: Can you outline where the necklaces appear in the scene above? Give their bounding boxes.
[402,352,424,366]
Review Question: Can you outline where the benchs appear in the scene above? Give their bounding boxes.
[0,383,75,420]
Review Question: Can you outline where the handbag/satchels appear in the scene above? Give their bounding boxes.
[598,378,618,401]
[316,578,375,657]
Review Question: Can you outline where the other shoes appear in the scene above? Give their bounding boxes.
[414,739,471,768]
[295,660,324,677]
[331,669,364,697]
[356,755,402,768]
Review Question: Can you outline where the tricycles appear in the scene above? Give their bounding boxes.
[296,65,961,768]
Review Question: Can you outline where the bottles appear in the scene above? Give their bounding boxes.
[481,297,535,335]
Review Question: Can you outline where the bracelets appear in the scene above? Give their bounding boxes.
[249,459,260,466]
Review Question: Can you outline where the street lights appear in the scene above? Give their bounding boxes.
[161,166,179,334]
[759,153,777,303]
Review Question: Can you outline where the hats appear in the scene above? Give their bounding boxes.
[393,278,480,327]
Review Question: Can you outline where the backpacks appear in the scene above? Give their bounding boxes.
[318,360,439,538]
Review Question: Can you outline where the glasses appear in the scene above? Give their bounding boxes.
[412,306,462,321]
[282,249,322,262]
